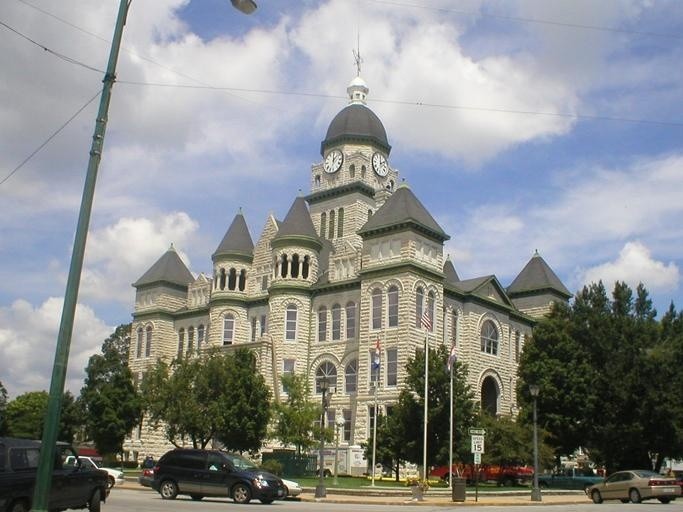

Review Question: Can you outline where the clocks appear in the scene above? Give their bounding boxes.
[322,148,343,174]
[371,151,388,177]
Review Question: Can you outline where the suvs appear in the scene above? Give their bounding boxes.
[150,449,286,505]
[0,437,109,511]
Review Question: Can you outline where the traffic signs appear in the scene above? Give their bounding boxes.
[470,435,484,455]
[467,427,488,437]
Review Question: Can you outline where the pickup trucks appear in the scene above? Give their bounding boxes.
[63,453,124,488]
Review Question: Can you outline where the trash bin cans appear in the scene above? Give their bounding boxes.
[452,477,466,502]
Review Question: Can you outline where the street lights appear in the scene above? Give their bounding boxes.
[527,378,541,501]
[331,416,345,486]
[314,376,330,498]
[28,0,257,512]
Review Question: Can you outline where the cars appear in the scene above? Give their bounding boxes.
[429,457,535,486]
[531,468,605,494]
[232,464,301,500]
[314,468,332,479]
[138,467,153,487]
[587,469,682,504]
[662,470,682,497]
